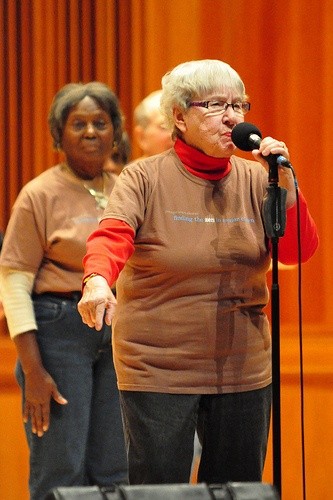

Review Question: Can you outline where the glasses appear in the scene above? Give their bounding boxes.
[189,100,251,113]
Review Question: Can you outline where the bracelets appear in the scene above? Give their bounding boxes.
[83,273,97,283]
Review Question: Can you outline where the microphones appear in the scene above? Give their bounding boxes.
[232,122,292,167]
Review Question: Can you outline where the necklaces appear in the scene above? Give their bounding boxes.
[65,164,110,209]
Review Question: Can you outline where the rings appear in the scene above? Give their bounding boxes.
[280,142,285,148]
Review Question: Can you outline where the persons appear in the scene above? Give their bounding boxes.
[132,89,203,480]
[0,82,130,500]
[78,60,319,484]
